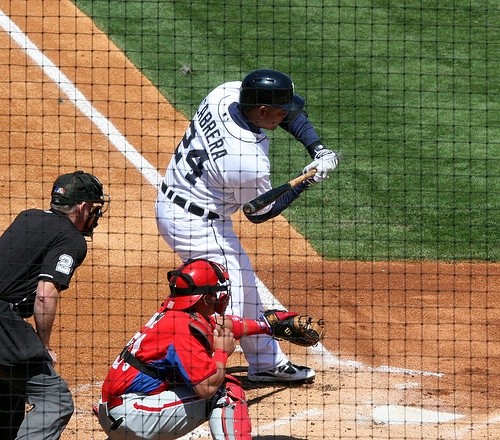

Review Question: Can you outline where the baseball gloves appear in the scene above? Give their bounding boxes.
[259,309,327,348]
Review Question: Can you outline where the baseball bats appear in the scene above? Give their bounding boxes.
[242,168,317,214]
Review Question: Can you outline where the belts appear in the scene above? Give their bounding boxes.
[107,397,123,411]
[161,180,220,220]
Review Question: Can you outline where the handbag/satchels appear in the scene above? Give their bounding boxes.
[0,300,53,366]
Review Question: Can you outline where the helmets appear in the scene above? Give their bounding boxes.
[51,170,105,206]
[162,257,232,317]
[238,69,307,113]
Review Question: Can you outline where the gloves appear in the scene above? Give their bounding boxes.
[301,148,338,185]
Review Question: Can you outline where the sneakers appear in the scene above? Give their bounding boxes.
[247,362,316,382]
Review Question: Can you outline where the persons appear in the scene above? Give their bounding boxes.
[155,70,340,381]
[98,257,326,440]
[0,171,111,440]
